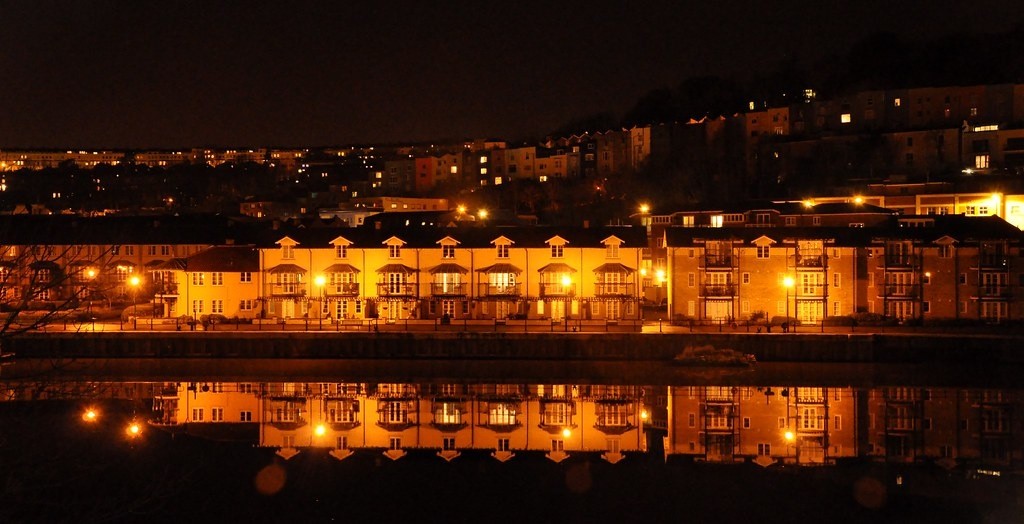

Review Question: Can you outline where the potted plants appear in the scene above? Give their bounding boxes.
[505,313,514,320]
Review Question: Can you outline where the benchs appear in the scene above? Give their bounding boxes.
[340,320,363,330]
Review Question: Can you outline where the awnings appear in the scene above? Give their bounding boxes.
[537,262,577,274]
[429,420,469,434]
[323,264,361,275]
[428,263,469,276]
[594,422,637,436]
[592,263,635,276]
[268,421,307,431]
[30,261,60,270]
[143,258,190,273]
[484,263,522,276]
[375,420,414,433]
[107,260,137,269]
[71,259,93,266]
[325,420,361,431]
[476,421,522,434]
[0,260,19,268]
[268,263,307,275]
[537,423,578,435]
[375,264,417,276]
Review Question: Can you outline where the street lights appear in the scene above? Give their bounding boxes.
[562,276,572,332]
[89,271,94,317]
[784,277,793,334]
[315,276,325,331]
[132,277,139,330]
